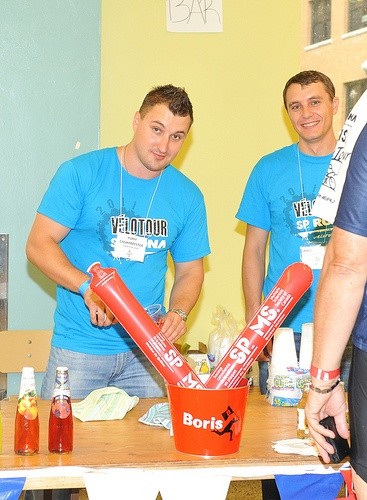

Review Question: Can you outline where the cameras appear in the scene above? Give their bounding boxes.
[319,415,350,464]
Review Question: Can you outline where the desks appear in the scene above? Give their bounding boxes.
[0,384,351,492]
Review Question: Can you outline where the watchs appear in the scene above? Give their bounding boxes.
[170,309,187,321]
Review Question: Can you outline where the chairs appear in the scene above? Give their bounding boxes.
[0,329,54,500]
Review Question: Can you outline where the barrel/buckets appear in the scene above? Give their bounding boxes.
[165,373,250,457]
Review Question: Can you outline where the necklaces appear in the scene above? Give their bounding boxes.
[124,145,128,171]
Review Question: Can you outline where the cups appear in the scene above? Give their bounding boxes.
[268,323,313,406]
[143,304,166,331]
[208,333,238,370]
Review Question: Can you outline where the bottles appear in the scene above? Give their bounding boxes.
[14,367,39,455]
[296,388,311,438]
[48,367,74,453]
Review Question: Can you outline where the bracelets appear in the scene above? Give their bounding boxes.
[78,279,91,297]
[311,365,340,381]
[309,377,341,394]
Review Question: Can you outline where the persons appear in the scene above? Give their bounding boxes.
[235,69,340,396]
[304,89,367,500]
[26,83,211,399]
